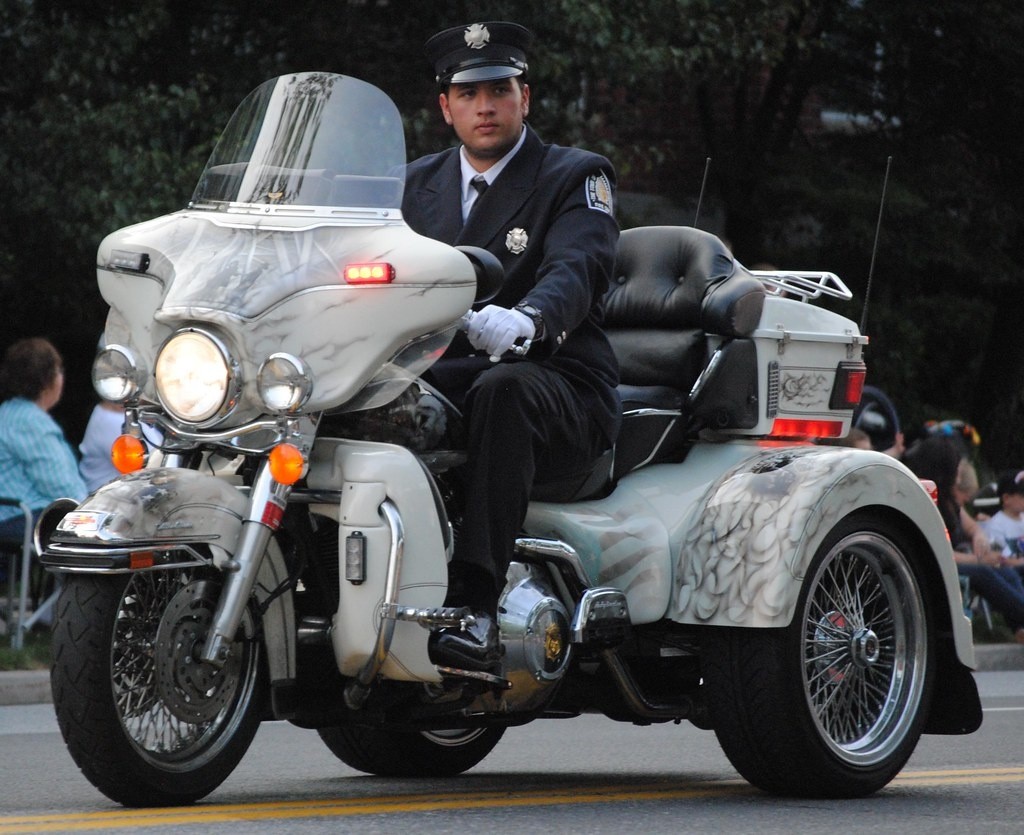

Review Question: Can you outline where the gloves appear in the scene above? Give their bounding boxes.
[468,304,535,357]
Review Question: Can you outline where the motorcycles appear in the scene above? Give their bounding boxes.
[33,68,986,808]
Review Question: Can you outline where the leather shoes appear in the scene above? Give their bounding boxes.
[433,613,505,679]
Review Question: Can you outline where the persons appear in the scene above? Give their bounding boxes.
[378,20,622,680]
[76,400,164,496]
[929,451,1024,644]
[0,336,90,643]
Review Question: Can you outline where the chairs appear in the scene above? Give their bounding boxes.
[0,496,62,648]
[961,576,992,635]
[603,227,763,479]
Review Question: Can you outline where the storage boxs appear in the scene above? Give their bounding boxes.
[719,292,870,440]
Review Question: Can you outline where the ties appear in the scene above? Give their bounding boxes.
[469,182,488,220]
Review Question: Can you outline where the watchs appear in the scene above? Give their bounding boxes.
[515,301,543,338]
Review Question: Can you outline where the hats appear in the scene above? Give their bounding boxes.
[424,21,536,82]
[997,469,1024,494]
